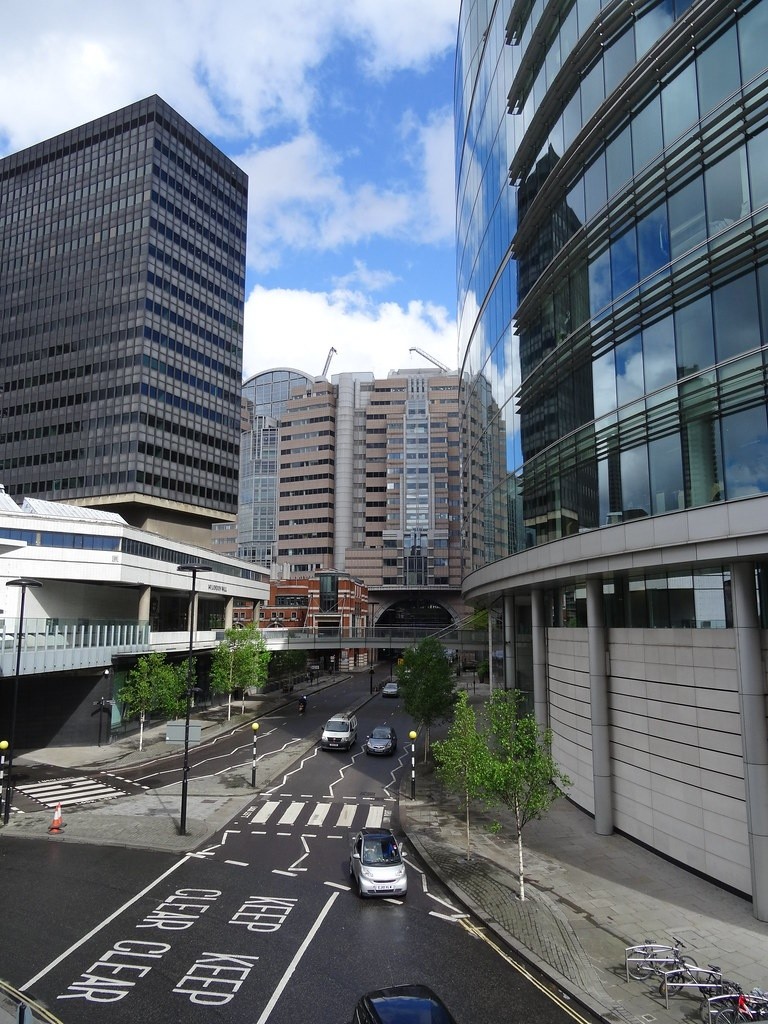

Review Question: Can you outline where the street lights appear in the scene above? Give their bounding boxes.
[385,609,396,682]
[177,563,214,836]
[3,577,44,826]
[367,601,380,669]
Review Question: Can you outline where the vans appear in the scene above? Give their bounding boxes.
[321,713,359,752]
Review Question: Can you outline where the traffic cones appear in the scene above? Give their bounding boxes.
[47,800,67,835]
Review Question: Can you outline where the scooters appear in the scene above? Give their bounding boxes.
[297,703,305,712]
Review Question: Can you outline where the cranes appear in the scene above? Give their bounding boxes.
[322,346,338,379]
[409,345,451,372]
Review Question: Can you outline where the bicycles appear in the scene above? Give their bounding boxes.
[624,933,768,1024]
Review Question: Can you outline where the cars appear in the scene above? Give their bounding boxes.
[352,984,458,1024]
[382,682,401,699]
[350,827,409,899]
[366,726,397,758]
